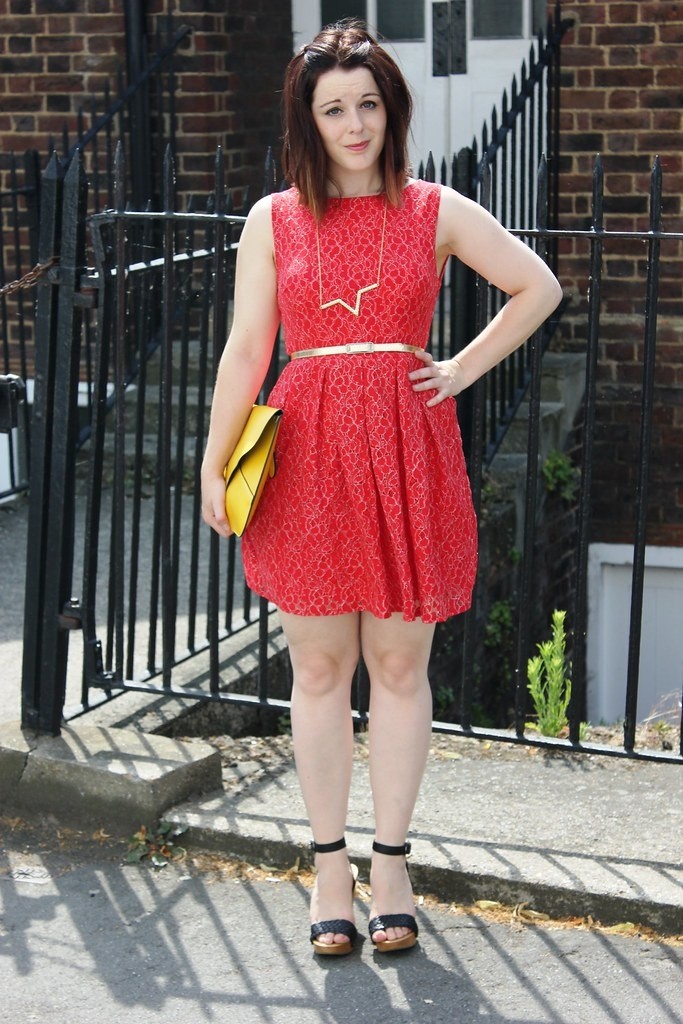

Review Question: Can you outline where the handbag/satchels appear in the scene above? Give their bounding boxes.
[223,404,284,538]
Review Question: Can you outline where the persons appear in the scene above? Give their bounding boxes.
[201,25,563,955]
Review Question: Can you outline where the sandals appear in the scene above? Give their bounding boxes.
[309,837,357,956]
[368,839,419,954]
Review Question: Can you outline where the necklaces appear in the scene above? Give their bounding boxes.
[316,198,388,315]
